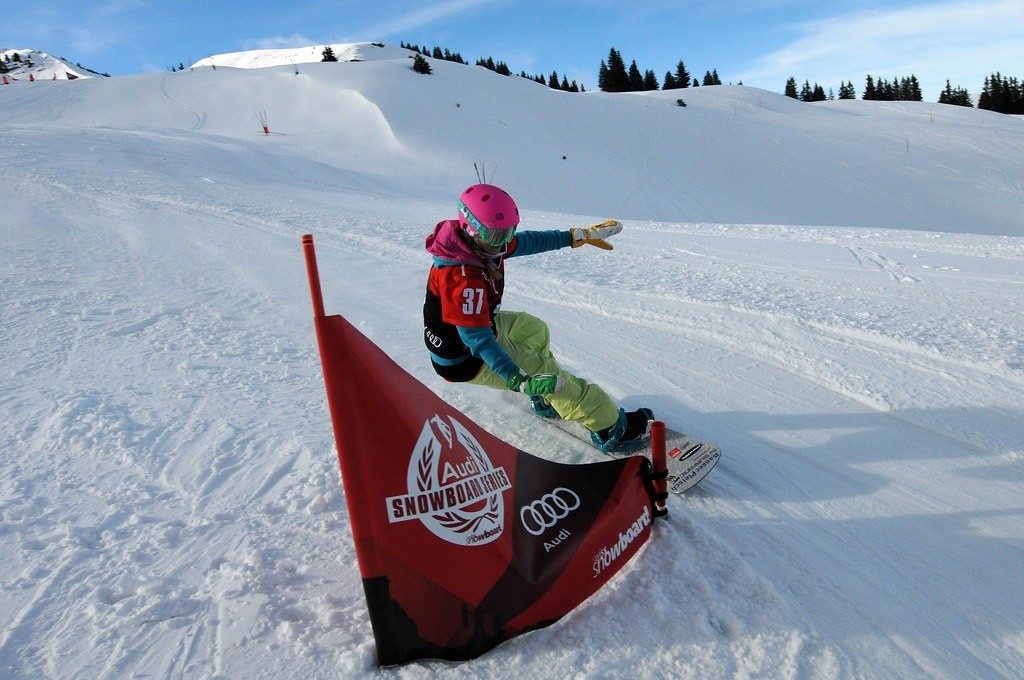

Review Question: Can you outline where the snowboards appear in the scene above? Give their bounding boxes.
[502,370,721,498]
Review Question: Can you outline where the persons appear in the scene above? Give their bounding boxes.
[424,184,654,453]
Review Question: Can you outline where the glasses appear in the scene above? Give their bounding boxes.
[482,226,517,247]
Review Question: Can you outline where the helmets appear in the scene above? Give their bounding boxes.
[456,183,521,238]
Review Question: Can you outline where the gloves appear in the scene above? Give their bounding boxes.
[571,220,622,250]
[504,366,563,396]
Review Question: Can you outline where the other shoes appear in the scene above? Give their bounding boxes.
[529,397,561,420]
[591,407,656,454]
